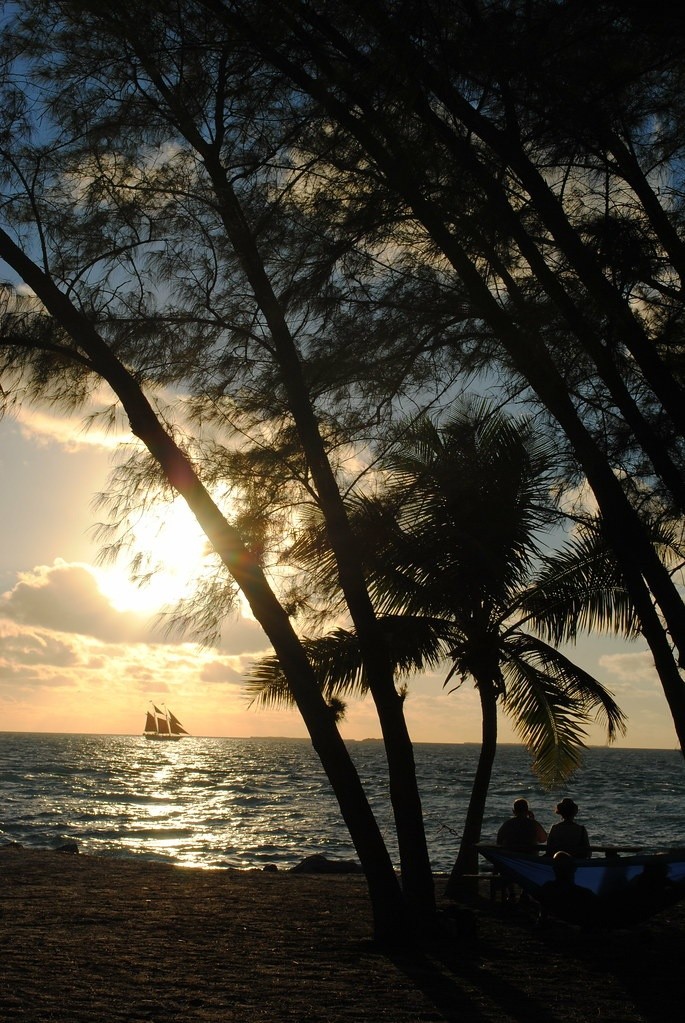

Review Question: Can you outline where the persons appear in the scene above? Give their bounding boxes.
[547,799,592,858]
[497,800,547,905]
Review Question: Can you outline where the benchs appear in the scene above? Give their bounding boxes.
[462,873,522,903]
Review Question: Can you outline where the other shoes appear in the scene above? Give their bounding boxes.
[507,892,516,903]
[520,888,529,902]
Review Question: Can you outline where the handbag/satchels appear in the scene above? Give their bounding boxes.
[575,826,587,858]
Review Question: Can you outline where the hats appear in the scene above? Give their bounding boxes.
[555,799,578,815]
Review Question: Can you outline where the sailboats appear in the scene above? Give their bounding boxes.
[141,701,190,742]
